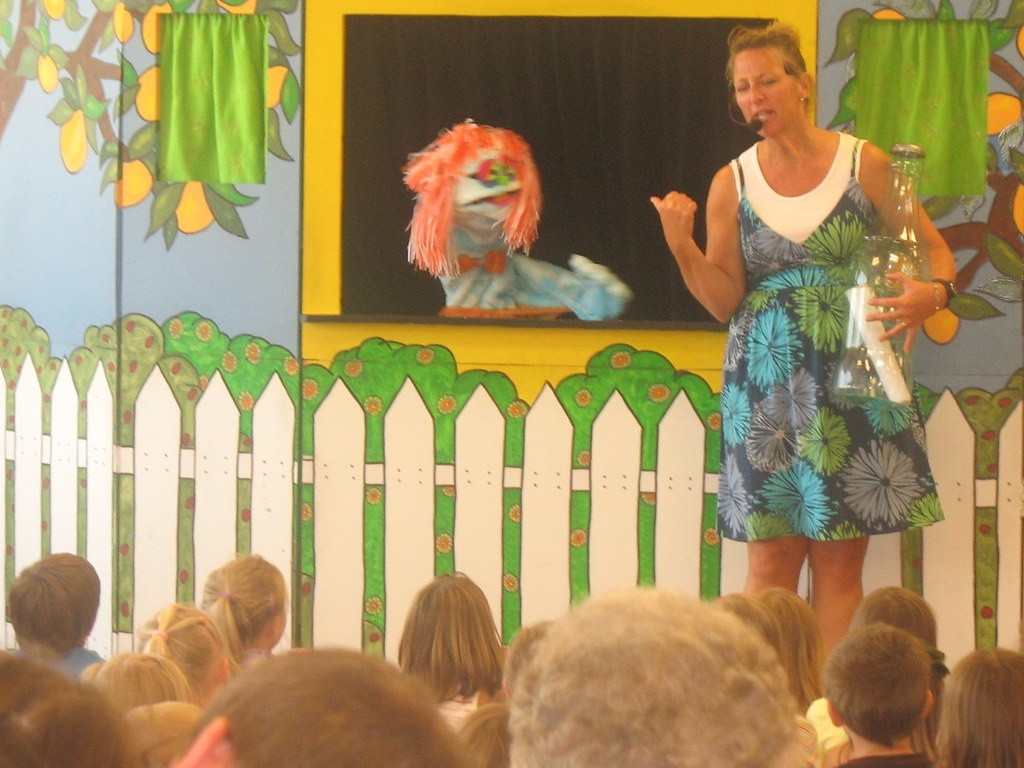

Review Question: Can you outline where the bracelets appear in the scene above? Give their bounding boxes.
[933,278,956,308]
[930,281,941,312]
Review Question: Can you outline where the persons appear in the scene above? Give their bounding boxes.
[824,623,935,768]
[202,555,289,667]
[398,572,503,736]
[81,604,230,768]
[939,647,1024,768]
[0,649,138,768]
[172,645,454,768]
[458,620,555,768]
[715,589,821,768]
[650,24,955,659]
[510,587,800,768]
[9,554,102,682]
[805,586,938,753]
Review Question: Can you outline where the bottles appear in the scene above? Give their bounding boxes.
[834,144,928,407]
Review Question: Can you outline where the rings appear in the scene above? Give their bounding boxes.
[900,322,907,329]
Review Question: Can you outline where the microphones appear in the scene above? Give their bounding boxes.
[728,102,763,131]
